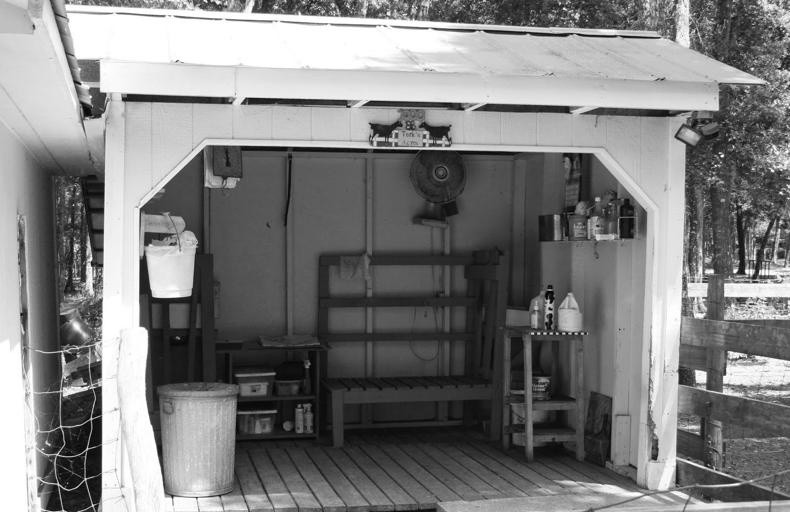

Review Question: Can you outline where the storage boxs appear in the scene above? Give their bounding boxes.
[275,378,303,397]
[233,367,277,399]
[236,410,278,435]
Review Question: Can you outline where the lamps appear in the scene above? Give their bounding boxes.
[676,110,720,148]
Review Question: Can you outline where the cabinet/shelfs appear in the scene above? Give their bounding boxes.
[214,338,327,441]
[501,324,590,463]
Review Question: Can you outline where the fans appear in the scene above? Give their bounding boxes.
[410,150,466,228]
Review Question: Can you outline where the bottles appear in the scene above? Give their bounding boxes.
[592,191,634,239]
[530,284,555,331]
[295,404,304,434]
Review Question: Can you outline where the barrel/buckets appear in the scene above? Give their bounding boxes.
[145,212,197,301]
[558,292,583,333]
[537,213,565,243]
[566,214,589,241]
[529,291,546,309]
[60,312,94,347]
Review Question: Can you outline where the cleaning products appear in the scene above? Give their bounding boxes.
[303,403,314,433]
[587,195,605,238]
[295,403,303,434]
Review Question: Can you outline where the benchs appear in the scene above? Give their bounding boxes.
[319,254,495,447]
